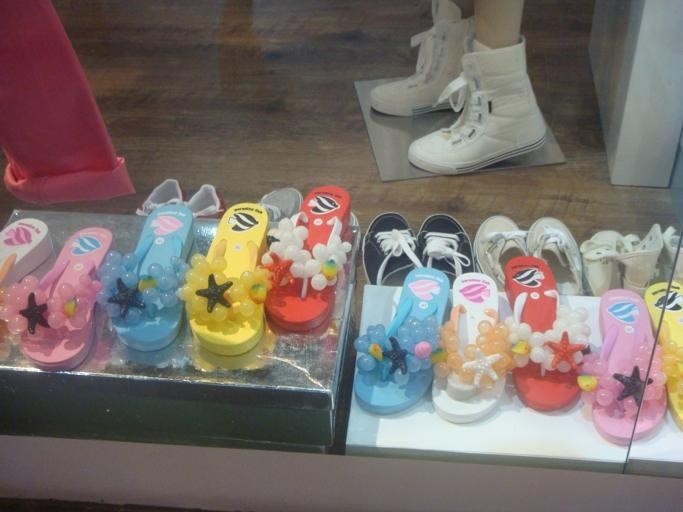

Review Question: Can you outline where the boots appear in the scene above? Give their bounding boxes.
[368,0,476,123]
[408,32,547,177]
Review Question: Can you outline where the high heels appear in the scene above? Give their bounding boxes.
[504,256,592,413]
[431,273,513,425]
[1,225,113,370]
[177,202,267,355]
[97,202,187,354]
[265,186,353,332]
[353,266,450,416]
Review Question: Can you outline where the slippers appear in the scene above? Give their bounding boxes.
[645,281,683,435]
[2,217,55,285]
[585,288,668,447]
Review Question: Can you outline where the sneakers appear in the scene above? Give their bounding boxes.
[665,226,683,285]
[416,214,476,287]
[527,217,582,295]
[361,211,422,288]
[581,223,664,299]
[473,215,526,290]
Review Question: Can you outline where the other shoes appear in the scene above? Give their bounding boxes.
[134,178,182,217]
[187,183,227,217]
[260,186,304,221]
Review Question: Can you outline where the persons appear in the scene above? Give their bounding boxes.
[366,0,549,178]
